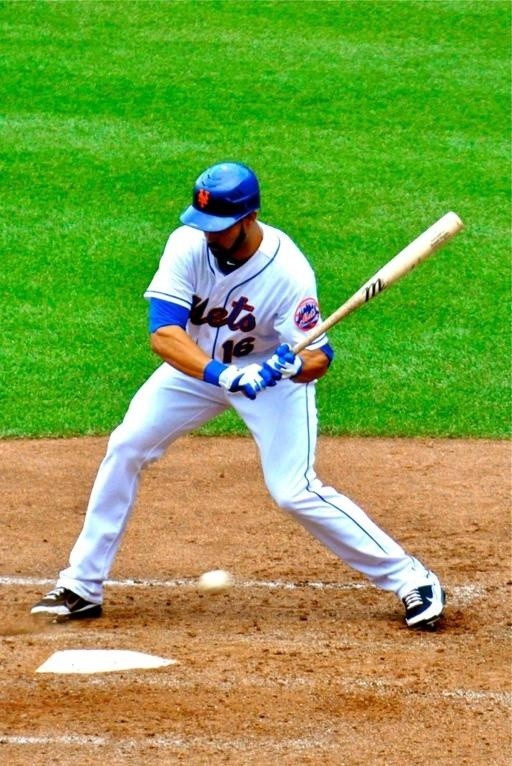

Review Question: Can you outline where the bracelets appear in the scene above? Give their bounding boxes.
[202,357,228,389]
[290,358,304,381]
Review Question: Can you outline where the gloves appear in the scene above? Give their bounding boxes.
[203,358,277,399]
[263,342,303,380]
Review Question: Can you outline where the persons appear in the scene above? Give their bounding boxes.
[27,159,447,630]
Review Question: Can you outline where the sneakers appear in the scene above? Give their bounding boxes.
[402,584,443,627]
[29,586,98,616]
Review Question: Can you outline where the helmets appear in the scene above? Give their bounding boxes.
[178,161,260,232]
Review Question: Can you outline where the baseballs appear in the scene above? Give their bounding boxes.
[201,570,231,590]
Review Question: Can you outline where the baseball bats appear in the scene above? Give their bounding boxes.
[293,207,464,359]
